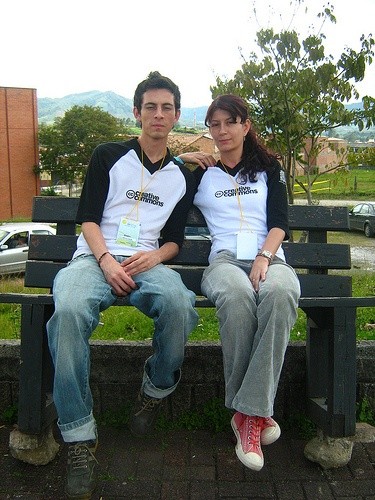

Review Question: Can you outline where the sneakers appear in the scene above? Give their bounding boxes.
[261,416,281,445]
[64,439,99,499]
[231,411,264,471]
[128,390,166,438]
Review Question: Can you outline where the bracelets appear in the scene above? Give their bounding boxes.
[98,251,110,268]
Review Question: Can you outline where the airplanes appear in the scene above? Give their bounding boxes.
[124,234,131,237]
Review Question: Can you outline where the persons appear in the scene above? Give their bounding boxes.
[174,94,301,472]
[46,71,197,498]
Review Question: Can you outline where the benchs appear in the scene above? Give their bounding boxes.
[0,196,375,438]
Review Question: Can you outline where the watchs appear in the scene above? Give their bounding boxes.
[256,251,273,266]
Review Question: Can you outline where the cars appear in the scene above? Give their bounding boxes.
[0,224,79,276]
[349,203,375,238]
